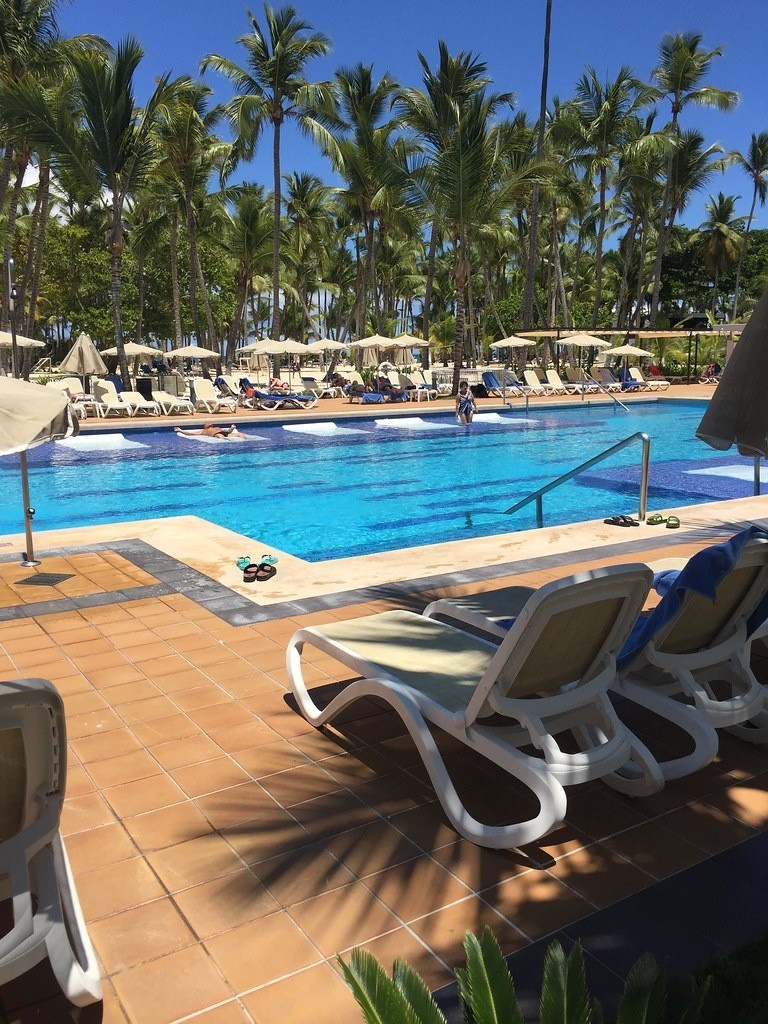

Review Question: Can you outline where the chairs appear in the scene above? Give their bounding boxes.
[0,678,103,1008]
[286,563,666,851]
[418,527,768,781]
[47,371,318,420]
[300,366,721,403]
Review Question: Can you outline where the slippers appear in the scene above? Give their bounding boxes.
[243,564,258,582]
[646,514,667,525]
[666,516,680,528]
[604,517,630,527]
[619,515,639,526]
[236,556,250,570]
[258,555,278,566]
[255,563,276,581]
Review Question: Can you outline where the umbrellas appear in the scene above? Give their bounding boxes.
[59,331,108,393]
[489,334,536,371]
[305,337,346,398]
[393,334,429,378]
[554,333,612,379]
[0,376,72,563]
[99,340,221,369]
[0,331,46,349]
[254,338,324,396]
[695,288,768,496]
[602,343,655,382]
[234,333,281,389]
[348,333,408,393]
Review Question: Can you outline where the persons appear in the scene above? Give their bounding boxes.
[702,361,721,384]
[644,363,661,377]
[245,385,261,398]
[140,359,167,374]
[456,381,478,425]
[293,354,302,379]
[174,423,244,439]
[314,357,319,362]
[332,373,406,405]
[270,378,294,390]
[341,349,347,366]
